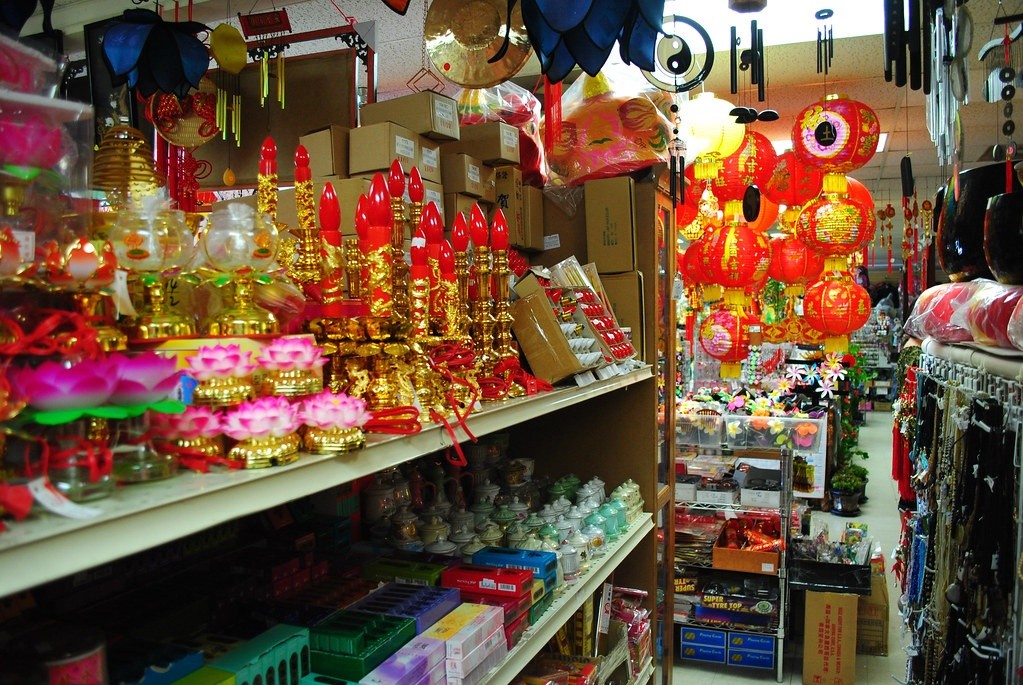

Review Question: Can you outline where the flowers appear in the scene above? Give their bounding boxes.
[658,350,855,447]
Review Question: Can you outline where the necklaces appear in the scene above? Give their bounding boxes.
[901,374,1023,685]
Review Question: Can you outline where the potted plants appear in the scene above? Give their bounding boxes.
[831,465,869,512]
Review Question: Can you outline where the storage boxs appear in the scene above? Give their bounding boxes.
[212,90,647,385]
[858,381,893,412]
[674,408,826,508]
[803,554,890,685]
[120,514,558,685]
[712,519,781,574]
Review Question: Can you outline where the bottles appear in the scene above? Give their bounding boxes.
[363,446,645,594]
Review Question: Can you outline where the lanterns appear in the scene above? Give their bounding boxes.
[679,92,880,378]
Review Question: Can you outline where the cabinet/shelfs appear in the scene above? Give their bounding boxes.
[673,447,794,682]
[0,364,656,685]
[507,161,691,685]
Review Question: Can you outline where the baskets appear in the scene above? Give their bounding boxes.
[151,76,219,147]
